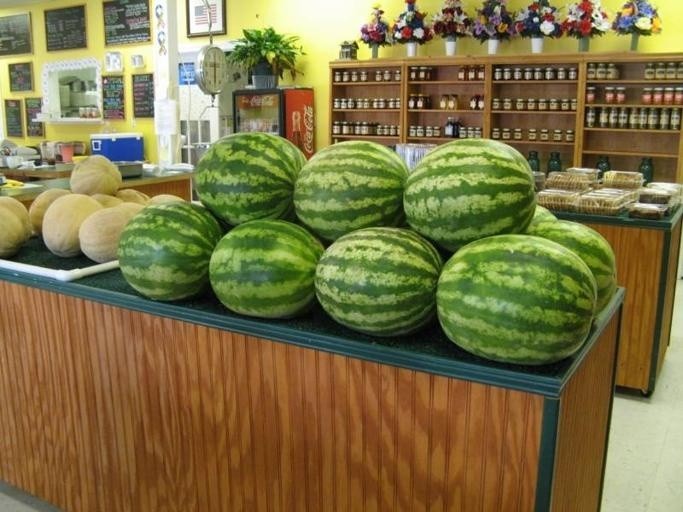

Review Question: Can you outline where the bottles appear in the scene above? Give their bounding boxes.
[290,111,302,150]
[526,151,539,171]
[444,117,462,138]
[547,152,560,176]
[639,157,653,185]
[595,156,609,178]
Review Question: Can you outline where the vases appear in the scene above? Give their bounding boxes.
[637,155,651,186]
[526,149,539,173]
[594,154,608,183]
[546,151,559,174]
[250,59,276,89]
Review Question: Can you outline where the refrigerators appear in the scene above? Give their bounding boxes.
[231,87,315,160]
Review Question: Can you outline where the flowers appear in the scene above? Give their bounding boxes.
[231,29,304,78]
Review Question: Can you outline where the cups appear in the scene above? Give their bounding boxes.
[60,143,73,162]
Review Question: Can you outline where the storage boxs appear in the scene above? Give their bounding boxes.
[91,133,144,161]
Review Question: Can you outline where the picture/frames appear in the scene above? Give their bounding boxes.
[183,1,226,40]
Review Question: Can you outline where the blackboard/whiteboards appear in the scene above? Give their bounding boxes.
[133,74,154,117]
[102,75,124,119]
[103,0,153,47]
[24,97,46,139]
[8,62,35,93]
[43,3,89,53]
[5,99,24,139]
[0,11,34,57]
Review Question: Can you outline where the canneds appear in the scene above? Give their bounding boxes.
[332,62,683,143]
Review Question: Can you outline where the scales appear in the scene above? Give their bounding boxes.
[188,44,229,152]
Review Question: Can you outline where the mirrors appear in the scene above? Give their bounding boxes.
[54,67,99,118]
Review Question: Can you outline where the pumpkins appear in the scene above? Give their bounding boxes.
[1,154,186,264]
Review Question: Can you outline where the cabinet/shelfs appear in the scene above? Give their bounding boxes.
[0,239,628,510]
[577,54,683,193]
[489,57,586,176]
[407,57,489,147]
[330,57,404,155]
[547,193,683,401]
[0,156,193,239]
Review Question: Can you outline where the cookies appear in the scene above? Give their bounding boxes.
[536,170,644,216]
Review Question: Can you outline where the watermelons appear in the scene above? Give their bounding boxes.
[117,131,617,366]
